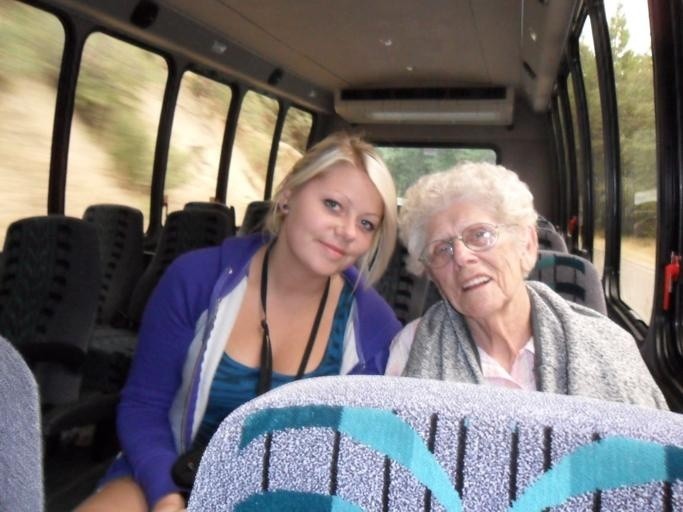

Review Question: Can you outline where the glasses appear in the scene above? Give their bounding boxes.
[418,221,515,269]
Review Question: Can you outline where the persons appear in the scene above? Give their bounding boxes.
[383,161,671,412]
[70,131,404,512]
